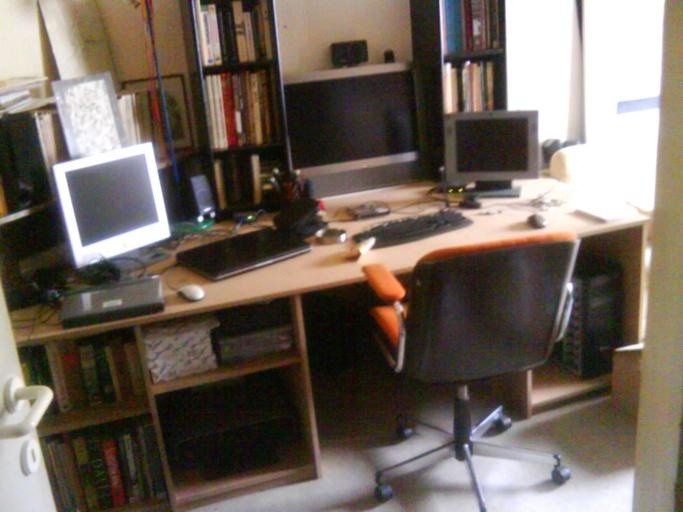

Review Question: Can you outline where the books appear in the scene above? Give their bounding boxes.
[19,334,169,512]
[439,1,496,114]
[0,73,172,218]
[194,0,282,208]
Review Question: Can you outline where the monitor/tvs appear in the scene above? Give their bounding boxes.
[444,110,539,197]
[51,142,172,270]
[283,63,425,182]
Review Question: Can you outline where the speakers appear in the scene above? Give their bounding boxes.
[189,174,216,215]
[331,40,368,66]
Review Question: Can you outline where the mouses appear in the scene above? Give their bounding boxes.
[528,214,548,229]
[177,284,206,302]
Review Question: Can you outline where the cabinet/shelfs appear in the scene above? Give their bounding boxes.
[0,0,508,278]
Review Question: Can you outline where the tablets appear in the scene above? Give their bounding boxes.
[176,228,312,280]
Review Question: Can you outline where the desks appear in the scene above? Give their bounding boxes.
[6,170,652,512]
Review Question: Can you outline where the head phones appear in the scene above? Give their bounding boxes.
[7,279,66,330]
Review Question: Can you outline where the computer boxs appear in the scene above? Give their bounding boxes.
[549,265,618,381]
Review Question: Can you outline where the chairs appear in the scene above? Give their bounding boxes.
[355,229,586,510]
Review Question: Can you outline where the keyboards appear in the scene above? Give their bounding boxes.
[352,209,473,250]
